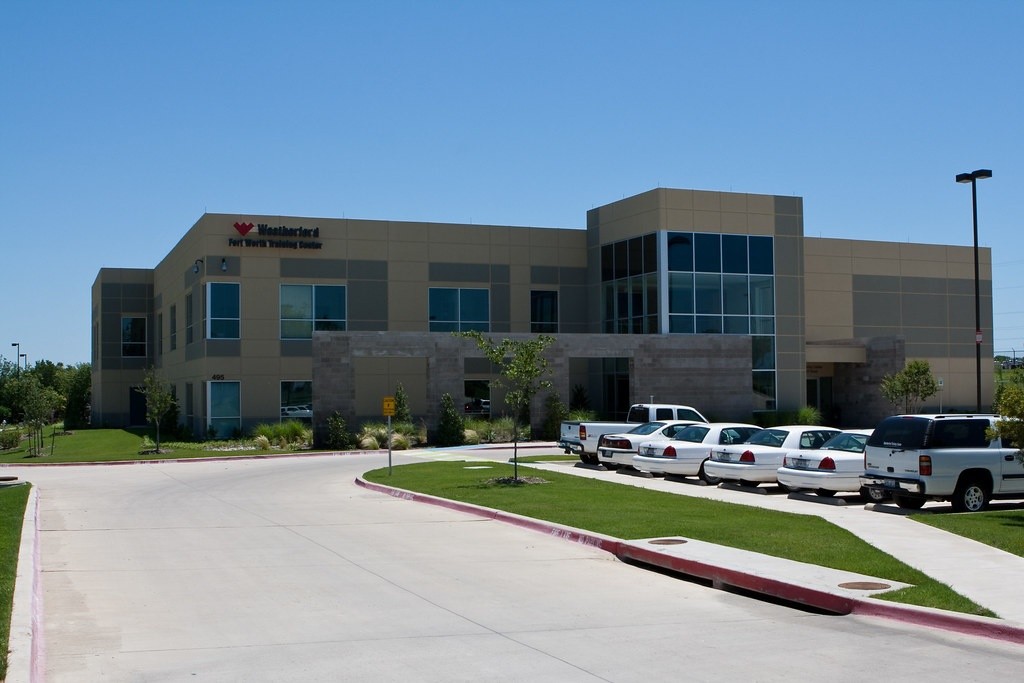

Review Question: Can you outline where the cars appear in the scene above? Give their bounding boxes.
[633,423,765,485]
[777,428,886,503]
[704,425,861,492]
[598,420,709,472]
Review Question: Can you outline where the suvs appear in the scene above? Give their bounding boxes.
[859,413,1024,512]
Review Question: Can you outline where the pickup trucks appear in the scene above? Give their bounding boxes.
[556,404,710,464]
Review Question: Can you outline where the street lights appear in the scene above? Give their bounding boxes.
[20,354,28,368]
[11,343,20,429]
[956,169,992,414]
